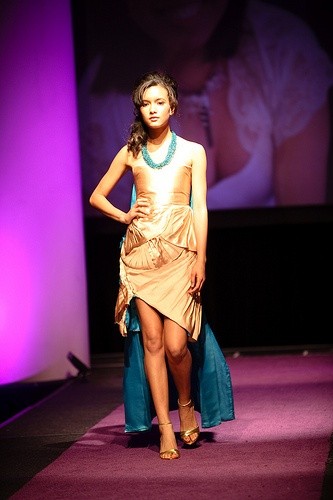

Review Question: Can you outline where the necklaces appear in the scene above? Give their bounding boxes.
[174,58,228,149]
[141,130,177,169]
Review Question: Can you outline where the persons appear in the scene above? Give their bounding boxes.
[89,75,208,461]
[71,0,333,218]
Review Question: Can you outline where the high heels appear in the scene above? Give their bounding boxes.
[158,423,180,459]
[175,398,200,446]
